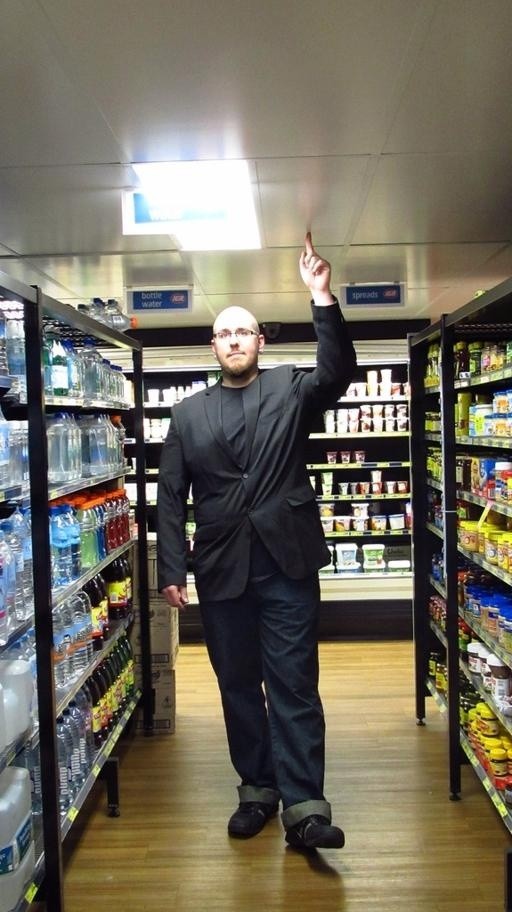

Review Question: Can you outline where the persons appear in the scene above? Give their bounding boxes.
[153,228,361,853]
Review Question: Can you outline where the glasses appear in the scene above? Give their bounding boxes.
[212,330,260,340]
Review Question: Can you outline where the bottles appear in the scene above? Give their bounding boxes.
[0,295,135,816]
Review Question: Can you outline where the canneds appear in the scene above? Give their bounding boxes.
[424,339,512,813]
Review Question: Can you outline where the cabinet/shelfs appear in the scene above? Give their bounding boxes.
[117,358,411,607]
[2,270,153,906]
[406,274,512,907]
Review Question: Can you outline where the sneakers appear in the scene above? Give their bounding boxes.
[228,801,279,840]
[285,815,345,849]
